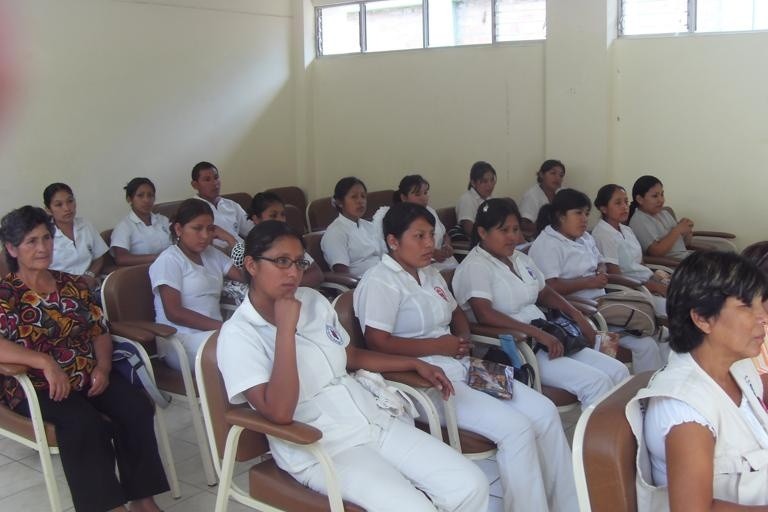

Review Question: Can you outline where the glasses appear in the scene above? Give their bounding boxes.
[256,256,310,271]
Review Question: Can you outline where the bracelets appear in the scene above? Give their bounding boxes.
[85,271,96,278]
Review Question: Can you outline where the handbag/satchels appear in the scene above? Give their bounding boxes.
[468,270,670,399]
[112,341,172,410]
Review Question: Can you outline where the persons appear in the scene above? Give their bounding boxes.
[147,198,245,373]
[0,205,170,512]
[110,177,175,266]
[216,220,489,512]
[739,240,768,409]
[321,159,696,512]
[191,162,256,249]
[625,252,767,511]
[230,191,324,289]
[44,183,110,304]
